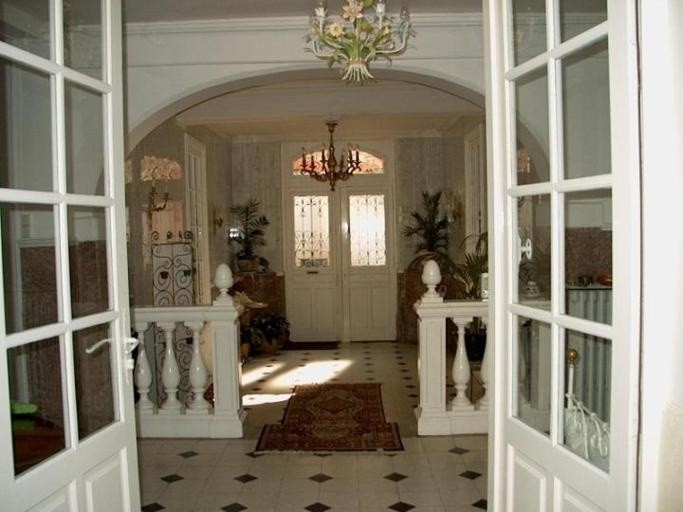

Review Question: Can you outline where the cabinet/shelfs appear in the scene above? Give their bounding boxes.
[516,297,550,433]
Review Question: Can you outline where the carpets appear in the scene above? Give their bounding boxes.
[254,384,404,453]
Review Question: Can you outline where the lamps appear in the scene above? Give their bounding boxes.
[139,154,183,213]
[299,120,361,193]
[302,0,417,85]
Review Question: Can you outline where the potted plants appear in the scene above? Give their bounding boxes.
[454,249,486,362]
[240,310,289,362]
[228,195,269,272]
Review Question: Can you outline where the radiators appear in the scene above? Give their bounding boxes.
[565,287,610,418]
